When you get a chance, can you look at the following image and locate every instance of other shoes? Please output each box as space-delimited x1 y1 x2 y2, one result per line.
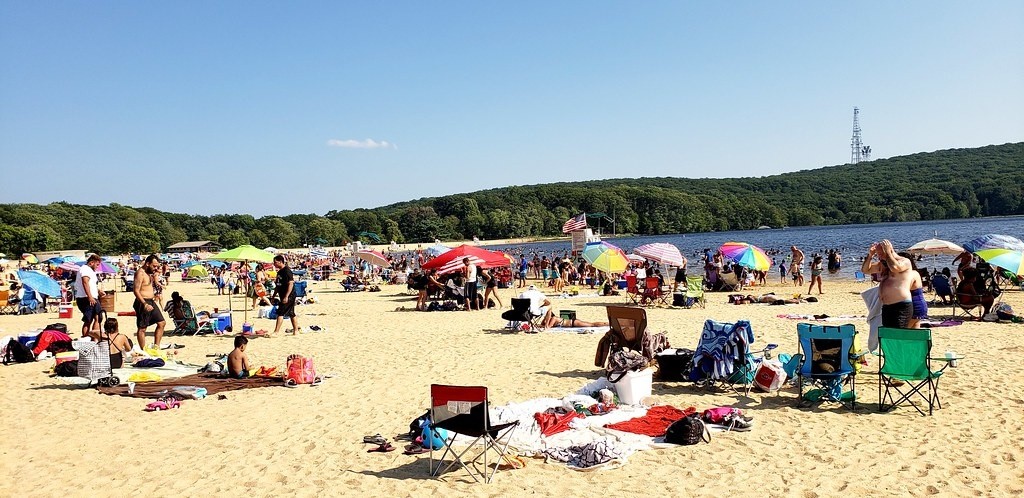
363 433 387 443
394 433 411 441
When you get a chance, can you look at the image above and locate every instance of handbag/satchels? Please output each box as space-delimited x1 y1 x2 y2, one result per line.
703 408 742 423
607 363 654 405
54 360 78 376
284 354 317 384
754 360 787 392
663 413 712 445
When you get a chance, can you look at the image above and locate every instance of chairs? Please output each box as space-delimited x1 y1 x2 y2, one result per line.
680 319 778 398
428 384 520 484
953 293 983 322
295 281 307 306
594 305 671 367
872 327 966 416
339 281 358 292
932 275 955 306
48 289 63 314
121 277 133 292
778 323 869 414
164 300 219 337
996 267 1024 290
684 276 705 308
0 290 39 315
502 298 542 333
626 276 671 305
719 271 740 292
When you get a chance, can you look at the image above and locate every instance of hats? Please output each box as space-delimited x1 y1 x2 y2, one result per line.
528 285 537 290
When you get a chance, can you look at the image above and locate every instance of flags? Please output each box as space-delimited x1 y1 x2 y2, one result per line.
562 212 586 233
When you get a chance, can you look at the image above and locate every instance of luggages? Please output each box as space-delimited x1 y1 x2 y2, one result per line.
72 309 120 387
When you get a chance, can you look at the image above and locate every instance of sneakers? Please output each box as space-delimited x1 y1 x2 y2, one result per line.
722 412 754 431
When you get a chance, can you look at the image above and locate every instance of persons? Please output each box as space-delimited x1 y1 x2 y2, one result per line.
75 253 107 338
0 259 139 314
157 244 287 334
85 311 265 380
288 238 1024 335
134 256 166 349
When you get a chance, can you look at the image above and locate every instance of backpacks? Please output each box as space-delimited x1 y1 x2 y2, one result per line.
3 340 35 365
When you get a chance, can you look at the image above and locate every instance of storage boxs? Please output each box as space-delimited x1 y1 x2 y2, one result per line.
613 280 627 290
56 351 79 365
58 302 73 318
673 292 692 306
17 331 43 348
585 277 598 285
610 367 652 405
211 312 231 332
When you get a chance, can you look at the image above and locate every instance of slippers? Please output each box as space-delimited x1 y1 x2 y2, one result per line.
368 442 395 452
285 379 299 388
404 443 431 454
311 374 323 385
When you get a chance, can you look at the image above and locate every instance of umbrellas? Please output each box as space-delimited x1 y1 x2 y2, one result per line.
582 241 629 274
633 242 683 267
422 243 515 288
717 241 773 271
905 234 1024 277
17 252 120 297
354 248 393 269
206 245 287 323
